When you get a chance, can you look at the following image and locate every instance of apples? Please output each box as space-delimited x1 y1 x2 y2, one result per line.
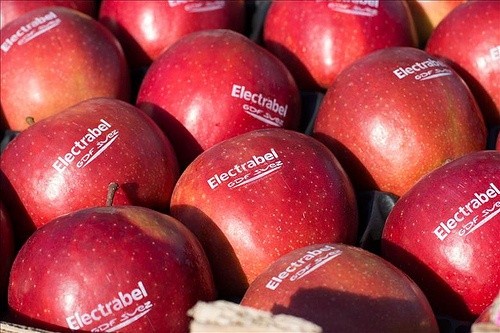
0 0 500 333
